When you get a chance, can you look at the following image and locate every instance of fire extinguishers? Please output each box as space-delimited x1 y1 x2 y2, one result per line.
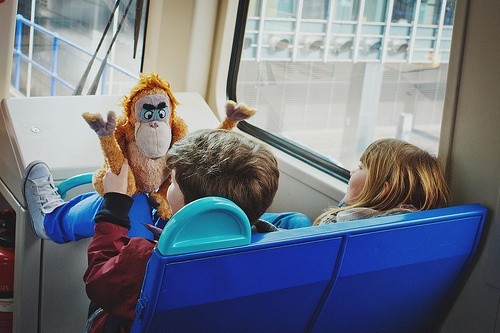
1 210 13 333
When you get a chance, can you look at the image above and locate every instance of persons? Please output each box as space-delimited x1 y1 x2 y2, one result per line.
21 130 281 333
252 138 449 238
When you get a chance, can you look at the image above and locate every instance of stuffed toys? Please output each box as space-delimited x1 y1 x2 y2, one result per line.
82 76 259 197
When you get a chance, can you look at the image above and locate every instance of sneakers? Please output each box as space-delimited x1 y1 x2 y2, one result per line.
21 160 65 240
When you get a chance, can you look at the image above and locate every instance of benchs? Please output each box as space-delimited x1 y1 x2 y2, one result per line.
129 196 488 333
